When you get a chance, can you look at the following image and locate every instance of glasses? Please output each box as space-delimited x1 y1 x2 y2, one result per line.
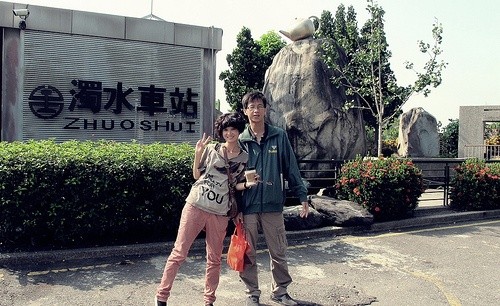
247 105 264 111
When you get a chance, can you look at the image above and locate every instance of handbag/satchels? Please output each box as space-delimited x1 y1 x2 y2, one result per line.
230 187 244 217
227 222 248 272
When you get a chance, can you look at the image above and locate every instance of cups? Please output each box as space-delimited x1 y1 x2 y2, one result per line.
245 170 257 186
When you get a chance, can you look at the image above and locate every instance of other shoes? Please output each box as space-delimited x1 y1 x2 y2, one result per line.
155 295 167 306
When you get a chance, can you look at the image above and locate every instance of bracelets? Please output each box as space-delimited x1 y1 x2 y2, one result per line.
244 182 251 189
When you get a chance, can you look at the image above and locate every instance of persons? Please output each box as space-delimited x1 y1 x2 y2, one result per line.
231 91 309 306
156 113 259 306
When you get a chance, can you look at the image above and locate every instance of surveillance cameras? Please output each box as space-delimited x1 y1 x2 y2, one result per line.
13 9 29 16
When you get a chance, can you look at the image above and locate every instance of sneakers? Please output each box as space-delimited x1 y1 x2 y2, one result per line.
246 296 259 306
270 293 298 306
